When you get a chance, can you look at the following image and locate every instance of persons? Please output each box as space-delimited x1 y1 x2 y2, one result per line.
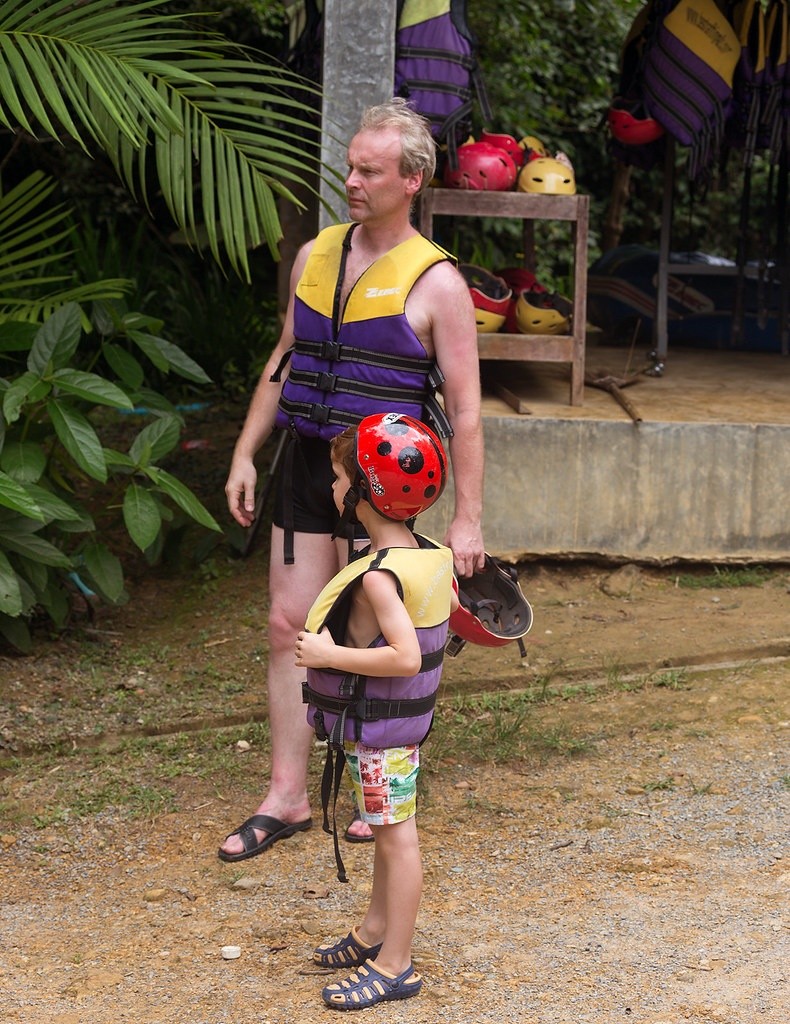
215 98 417 862
293 413 455 1010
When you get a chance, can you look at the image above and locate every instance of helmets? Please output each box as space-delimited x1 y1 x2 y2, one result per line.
453 263 572 334
427 128 577 197
609 99 663 145
450 550 532 649
356 413 448 522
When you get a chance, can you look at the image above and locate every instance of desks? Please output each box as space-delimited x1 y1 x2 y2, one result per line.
417 185 591 407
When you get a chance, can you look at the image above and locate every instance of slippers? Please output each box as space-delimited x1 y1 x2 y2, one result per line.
219 811 313 863
344 803 378 841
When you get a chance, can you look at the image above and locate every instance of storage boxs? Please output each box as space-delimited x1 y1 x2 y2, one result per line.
667 249 738 350
742 259 782 353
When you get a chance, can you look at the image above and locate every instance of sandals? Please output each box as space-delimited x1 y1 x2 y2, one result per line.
322 959 423 1008
313 925 384 968
